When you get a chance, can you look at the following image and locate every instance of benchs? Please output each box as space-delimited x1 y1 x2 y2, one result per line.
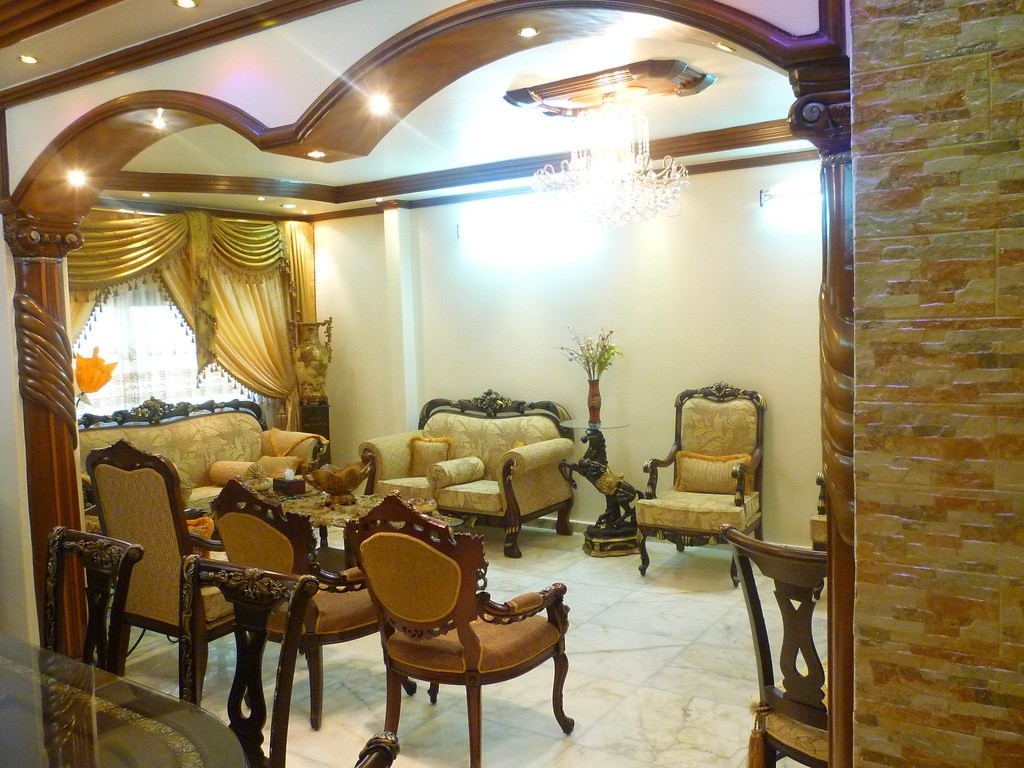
358 389 576 558
78 397 329 513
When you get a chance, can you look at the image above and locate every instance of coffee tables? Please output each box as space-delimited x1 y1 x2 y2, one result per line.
265 492 464 547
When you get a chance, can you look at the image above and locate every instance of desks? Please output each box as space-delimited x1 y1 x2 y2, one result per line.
0 635 246 768
558 419 645 558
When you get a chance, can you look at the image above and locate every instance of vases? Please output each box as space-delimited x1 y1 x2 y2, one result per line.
587 380 601 423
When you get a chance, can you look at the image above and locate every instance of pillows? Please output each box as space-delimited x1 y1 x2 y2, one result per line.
406 435 454 477
674 450 752 495
255 454 302 478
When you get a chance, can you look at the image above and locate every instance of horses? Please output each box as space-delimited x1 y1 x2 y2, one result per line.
558 424 644 530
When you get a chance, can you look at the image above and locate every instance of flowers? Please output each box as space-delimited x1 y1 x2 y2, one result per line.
555 325 624 380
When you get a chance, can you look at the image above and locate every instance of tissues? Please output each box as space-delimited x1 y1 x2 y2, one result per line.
273 468 306 495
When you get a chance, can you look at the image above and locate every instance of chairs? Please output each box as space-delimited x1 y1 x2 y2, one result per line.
210 478 379 730
85 438 234 708
636 381 767 587
721 524 828 768
41 526 144 678
343 492 575 768
